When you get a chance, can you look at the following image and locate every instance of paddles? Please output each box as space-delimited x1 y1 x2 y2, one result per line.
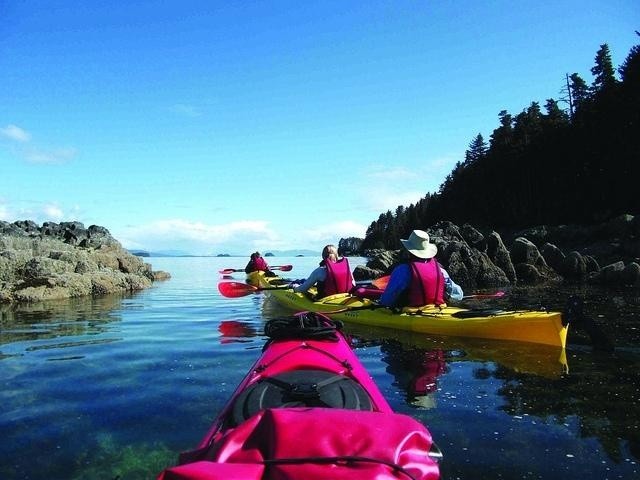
218 275 392 298
218 265 293 274
320 292 504 314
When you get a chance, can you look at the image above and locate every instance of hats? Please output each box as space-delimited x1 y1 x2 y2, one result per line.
400 230 438 259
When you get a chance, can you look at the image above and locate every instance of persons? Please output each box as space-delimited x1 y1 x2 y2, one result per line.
244 251 270 274
380 344 448 410
292 244 356 297
380 229 464 307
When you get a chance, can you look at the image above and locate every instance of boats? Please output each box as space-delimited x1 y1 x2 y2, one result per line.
260 295 571 378
247 270 290 289
157 310 445 478
258 275 572 349
